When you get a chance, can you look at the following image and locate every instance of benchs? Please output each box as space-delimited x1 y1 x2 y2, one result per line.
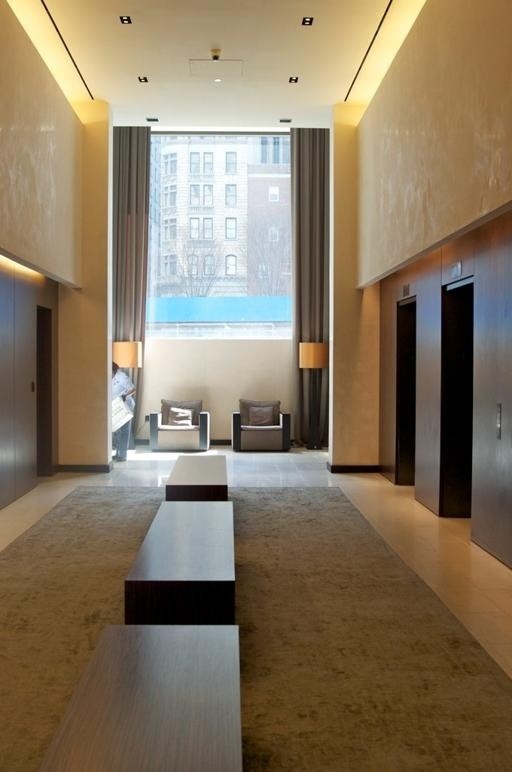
123 499 237 625
42 623 241 772
163 455 229 501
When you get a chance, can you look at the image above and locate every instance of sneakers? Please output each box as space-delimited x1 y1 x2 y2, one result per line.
112 454 127 462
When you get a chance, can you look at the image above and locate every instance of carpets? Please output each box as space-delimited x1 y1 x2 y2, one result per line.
2 485 509 772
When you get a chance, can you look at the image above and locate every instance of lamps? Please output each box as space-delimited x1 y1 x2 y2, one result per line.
113 341 142 378
298 342 328 450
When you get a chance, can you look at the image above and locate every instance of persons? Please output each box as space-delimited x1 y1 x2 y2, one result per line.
110 361 138 463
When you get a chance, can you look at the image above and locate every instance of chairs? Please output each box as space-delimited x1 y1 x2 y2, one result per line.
149 398 291 451
